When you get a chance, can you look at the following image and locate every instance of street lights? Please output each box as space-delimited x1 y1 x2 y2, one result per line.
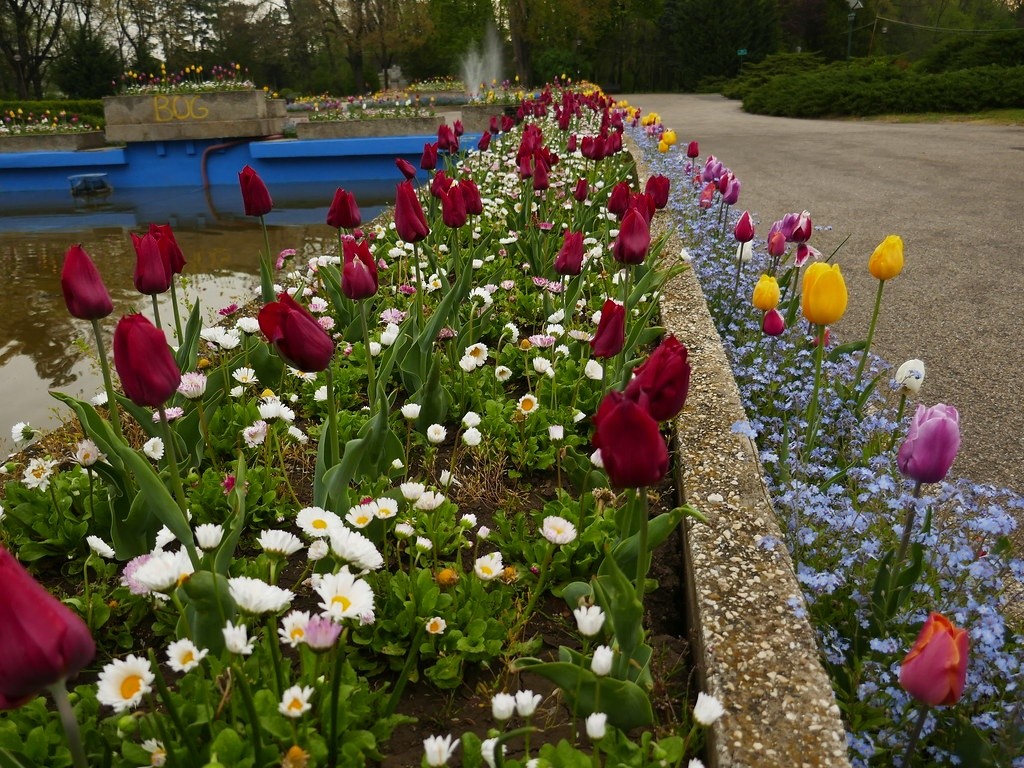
847 13 856 57
